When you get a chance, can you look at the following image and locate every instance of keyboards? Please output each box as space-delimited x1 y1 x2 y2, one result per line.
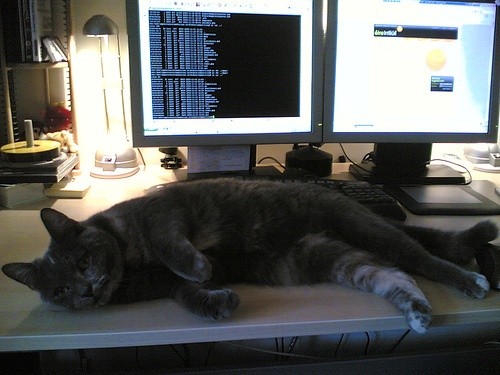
313 179 406 221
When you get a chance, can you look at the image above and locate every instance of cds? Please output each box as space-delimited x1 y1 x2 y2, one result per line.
0 139 68 169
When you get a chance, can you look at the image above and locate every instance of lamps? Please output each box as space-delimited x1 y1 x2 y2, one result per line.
84 15 139 179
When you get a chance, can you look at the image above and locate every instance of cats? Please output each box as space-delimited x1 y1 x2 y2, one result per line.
1 176 500 335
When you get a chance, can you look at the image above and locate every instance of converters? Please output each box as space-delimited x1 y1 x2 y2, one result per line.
251 166 283 182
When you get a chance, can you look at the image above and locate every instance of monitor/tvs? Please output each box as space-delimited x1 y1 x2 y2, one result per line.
125 0 323 180
324 0 499 184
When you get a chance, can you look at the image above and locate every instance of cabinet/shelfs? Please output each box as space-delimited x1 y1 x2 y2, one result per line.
0 3 83 190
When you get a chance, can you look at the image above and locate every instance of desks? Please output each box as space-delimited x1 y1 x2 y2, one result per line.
1 161 500 354
